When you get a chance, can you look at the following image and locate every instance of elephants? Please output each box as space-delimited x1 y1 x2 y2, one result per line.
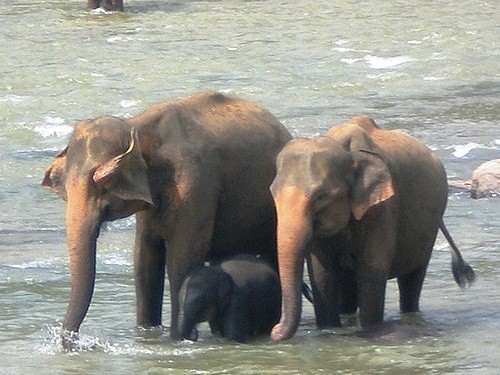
267 115 476 342
43 92 293 348
172 249 284 341
